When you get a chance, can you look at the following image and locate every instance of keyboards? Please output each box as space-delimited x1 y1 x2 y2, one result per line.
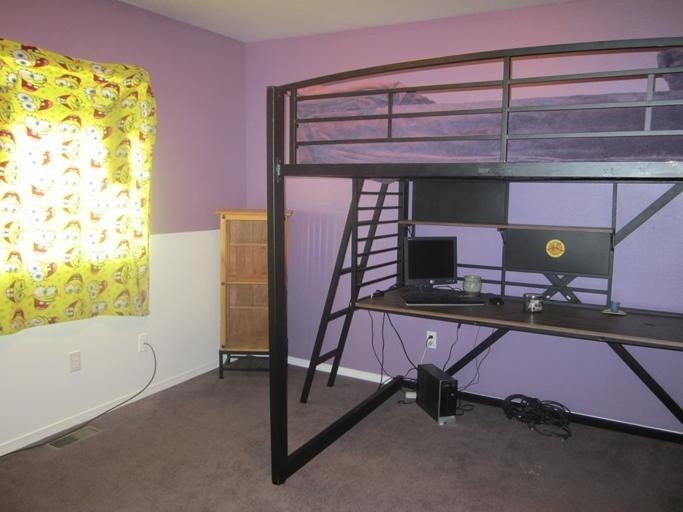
401 295 485 307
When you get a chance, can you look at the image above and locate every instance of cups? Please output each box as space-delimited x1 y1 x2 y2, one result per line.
463 274 481 295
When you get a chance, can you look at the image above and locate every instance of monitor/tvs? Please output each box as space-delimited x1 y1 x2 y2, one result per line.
403 236 457 294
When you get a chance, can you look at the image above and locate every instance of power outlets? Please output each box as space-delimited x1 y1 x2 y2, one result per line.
427 331 437 349
139 334 151 352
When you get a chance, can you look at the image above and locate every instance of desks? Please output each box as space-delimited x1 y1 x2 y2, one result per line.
356 286 683 354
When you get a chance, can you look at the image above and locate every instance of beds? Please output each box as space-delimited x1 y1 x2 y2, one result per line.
267 39 683 485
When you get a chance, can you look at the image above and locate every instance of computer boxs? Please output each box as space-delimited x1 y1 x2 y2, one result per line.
416 363 458 426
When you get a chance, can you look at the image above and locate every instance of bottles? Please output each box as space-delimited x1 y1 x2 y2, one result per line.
524 293 544 313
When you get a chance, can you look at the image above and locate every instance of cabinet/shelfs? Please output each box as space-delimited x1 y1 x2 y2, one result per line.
213 207 294 382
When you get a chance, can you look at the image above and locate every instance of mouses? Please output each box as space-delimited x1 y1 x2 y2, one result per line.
489 296 503 306
